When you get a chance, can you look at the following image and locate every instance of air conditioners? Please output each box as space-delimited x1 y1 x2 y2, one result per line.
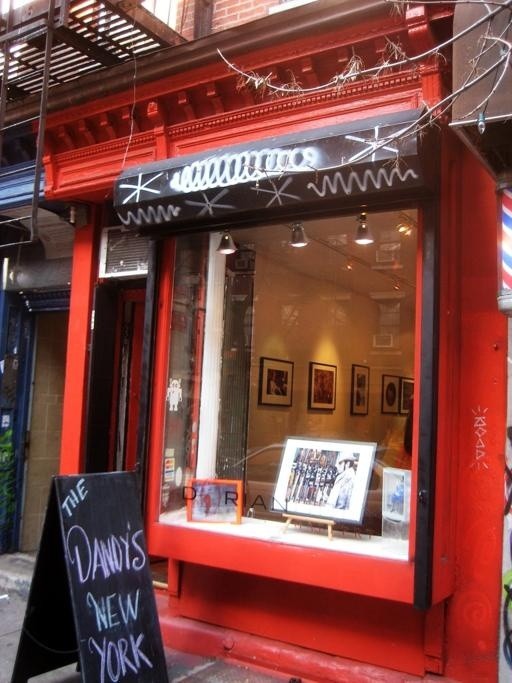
376 250 394 262
373 334 394 348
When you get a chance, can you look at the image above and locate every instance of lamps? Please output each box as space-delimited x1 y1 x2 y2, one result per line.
289 221 309 249
354 212 374 245
216 230 239 255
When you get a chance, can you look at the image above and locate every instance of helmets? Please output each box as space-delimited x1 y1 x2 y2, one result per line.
336 452 356 465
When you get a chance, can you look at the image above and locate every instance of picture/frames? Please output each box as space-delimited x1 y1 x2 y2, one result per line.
308 361 337 410
269 435 379 526
258 357 294 407
382 374 414 416
351 364 370 416
186 478 242 525
382 466 411 524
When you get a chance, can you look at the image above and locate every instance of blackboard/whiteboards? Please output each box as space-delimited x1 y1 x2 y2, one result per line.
54 469 168 683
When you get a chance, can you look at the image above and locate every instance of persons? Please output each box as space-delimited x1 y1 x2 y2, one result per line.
294 449 357 510
387 476 405 513
204 489 212 515
271 371 281 395
279 377 288 395
